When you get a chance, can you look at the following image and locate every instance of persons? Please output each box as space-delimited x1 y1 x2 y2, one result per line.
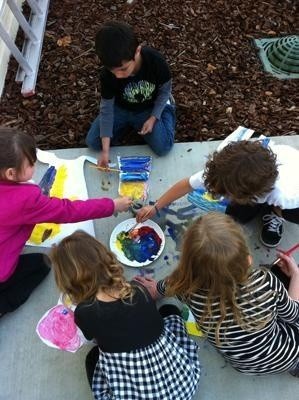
51 231 201 398
132 214 299 377
1 127 132 317
135 138 299 247
84 21 175 172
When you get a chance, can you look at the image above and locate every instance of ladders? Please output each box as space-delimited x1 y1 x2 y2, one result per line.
0 0 49 98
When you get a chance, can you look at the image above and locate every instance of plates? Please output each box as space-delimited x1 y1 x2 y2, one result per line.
109 216 167 268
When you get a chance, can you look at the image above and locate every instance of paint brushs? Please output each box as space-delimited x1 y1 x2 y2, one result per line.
125 202 158 236
88 164 126 173
130 200 141 208
272 243 299 266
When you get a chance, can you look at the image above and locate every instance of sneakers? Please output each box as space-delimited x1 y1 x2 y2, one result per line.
259 210 286 248
